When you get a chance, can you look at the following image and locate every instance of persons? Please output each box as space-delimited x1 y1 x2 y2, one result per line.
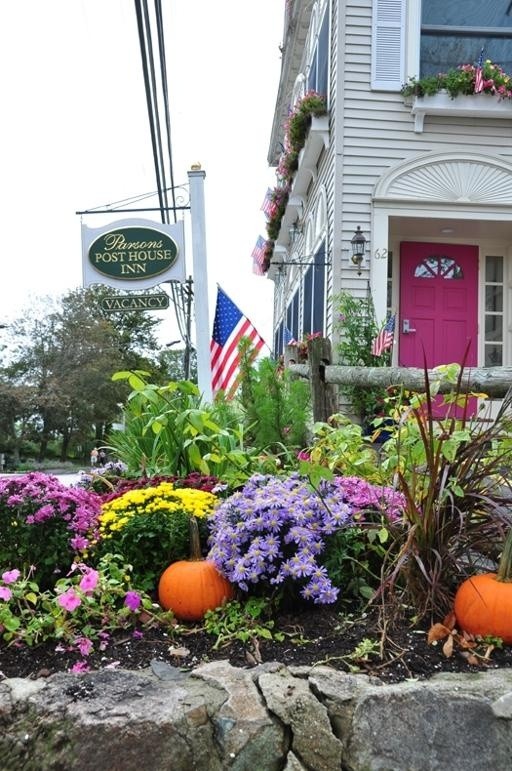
98 448 107 465
90 445 99 468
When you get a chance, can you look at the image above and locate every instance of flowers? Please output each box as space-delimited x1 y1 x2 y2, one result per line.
254 87 325 276
398 60 512 105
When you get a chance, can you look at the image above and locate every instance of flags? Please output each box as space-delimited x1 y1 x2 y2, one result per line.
369 314 395 357
209 280 271 407
473 46 486 95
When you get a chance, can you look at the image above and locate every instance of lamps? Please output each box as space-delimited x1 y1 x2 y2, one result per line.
350 224 367 276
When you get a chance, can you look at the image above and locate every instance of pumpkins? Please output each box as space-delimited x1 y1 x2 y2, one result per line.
455 534 512 647
158 517 235 622
